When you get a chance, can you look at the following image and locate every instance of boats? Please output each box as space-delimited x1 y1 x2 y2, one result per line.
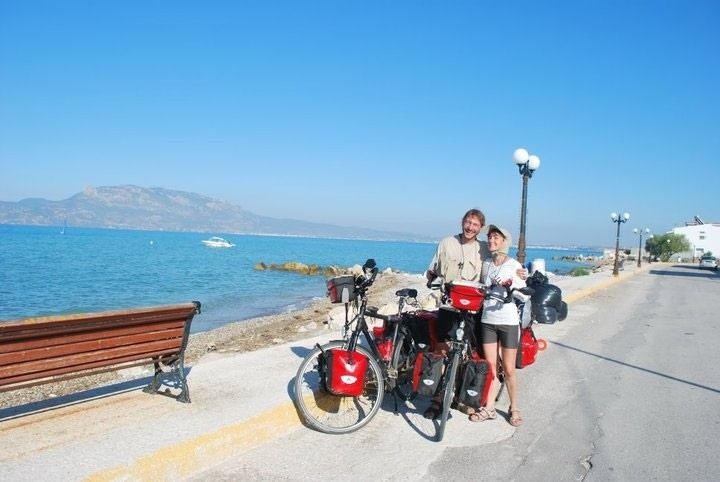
201 237 234 248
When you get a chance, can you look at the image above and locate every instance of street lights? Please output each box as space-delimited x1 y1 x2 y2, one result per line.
611 212 630 274
634 227 654 266
514 148 540 267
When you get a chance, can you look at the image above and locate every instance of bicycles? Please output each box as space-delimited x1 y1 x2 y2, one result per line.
430 272 522 441
295 258 430 435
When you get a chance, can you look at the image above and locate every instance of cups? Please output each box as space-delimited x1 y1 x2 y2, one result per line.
376 336 393 361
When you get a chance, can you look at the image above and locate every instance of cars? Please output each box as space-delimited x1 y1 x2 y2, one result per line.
698 255 717 270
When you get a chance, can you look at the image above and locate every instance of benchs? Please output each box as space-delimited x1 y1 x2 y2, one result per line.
0 298 204 405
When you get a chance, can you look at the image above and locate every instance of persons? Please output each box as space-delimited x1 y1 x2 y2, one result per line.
420 208 529 310
468 223 526 427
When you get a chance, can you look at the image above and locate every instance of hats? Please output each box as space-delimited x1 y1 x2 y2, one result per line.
485 224 512 258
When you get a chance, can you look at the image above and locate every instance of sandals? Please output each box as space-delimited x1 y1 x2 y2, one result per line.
507 406 523 427
469 408 498 423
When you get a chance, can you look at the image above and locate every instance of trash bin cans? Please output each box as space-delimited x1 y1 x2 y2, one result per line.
529 259 546 276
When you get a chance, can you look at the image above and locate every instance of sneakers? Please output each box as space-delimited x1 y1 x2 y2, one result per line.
423 407 439 420
451 401 475 416
